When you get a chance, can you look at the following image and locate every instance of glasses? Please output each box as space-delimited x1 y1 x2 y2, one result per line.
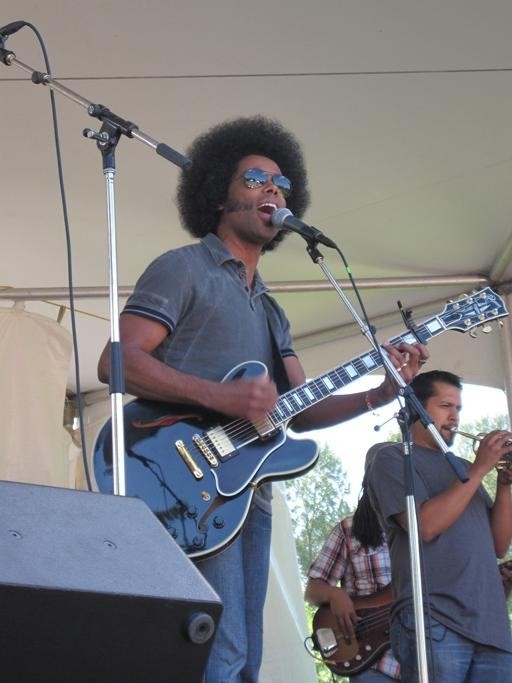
231 168 293 198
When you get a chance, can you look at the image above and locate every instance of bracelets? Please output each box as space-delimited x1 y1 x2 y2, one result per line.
365 389 375 411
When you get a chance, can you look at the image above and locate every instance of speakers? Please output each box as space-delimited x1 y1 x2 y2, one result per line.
0 480 224 683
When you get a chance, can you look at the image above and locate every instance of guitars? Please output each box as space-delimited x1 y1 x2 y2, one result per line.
93 286 508 562
311 562 511 676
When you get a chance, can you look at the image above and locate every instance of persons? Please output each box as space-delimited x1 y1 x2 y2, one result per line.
97 116 429 682
303 440 512 683
367 370 512 683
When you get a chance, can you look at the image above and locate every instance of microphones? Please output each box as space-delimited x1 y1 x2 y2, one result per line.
271 207 338 249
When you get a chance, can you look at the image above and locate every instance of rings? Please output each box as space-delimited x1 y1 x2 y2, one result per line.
396 367 402 371
418 359 425 363
401 362 407 367
